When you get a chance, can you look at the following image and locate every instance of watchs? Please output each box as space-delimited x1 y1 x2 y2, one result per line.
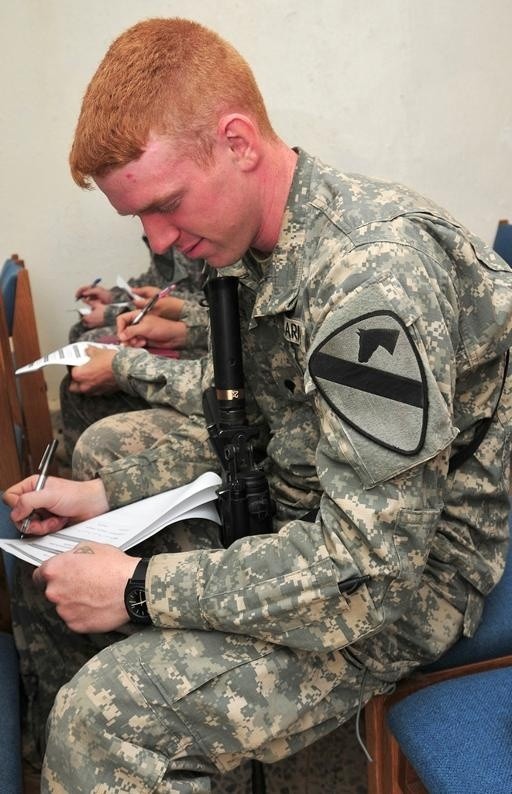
124 557 153 627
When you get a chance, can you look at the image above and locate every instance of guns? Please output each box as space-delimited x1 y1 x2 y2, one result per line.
202 275 276 792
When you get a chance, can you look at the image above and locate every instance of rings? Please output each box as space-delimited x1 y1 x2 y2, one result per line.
77 384 81 393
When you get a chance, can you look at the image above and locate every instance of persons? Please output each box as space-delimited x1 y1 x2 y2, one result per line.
56 235 215 482
0 17 512 794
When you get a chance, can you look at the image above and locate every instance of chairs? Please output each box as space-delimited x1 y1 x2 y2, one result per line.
358 218 512 794
0 254 59 791
